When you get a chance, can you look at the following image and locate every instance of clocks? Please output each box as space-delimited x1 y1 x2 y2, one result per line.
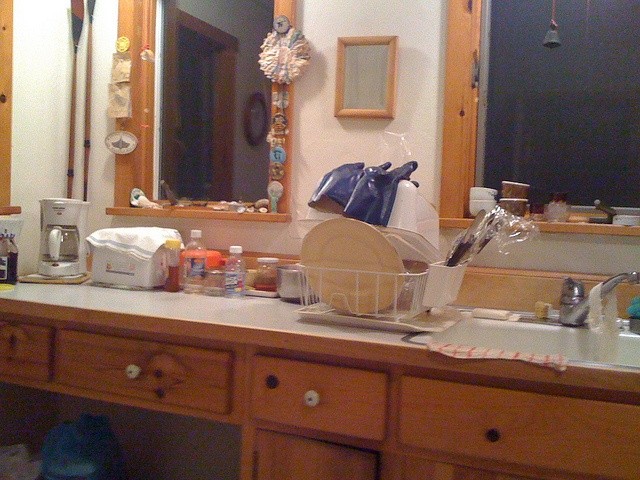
245 94 267 146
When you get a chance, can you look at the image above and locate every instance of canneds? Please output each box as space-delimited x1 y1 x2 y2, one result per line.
254 257 279 291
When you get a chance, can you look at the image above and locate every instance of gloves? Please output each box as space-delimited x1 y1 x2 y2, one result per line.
343 161 419 228
308 162 365 207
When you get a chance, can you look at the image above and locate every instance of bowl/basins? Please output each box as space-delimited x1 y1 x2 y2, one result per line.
277 266 318 302
470 187 496 201
468 201 497 219
500 200 527 218
500 181 529 199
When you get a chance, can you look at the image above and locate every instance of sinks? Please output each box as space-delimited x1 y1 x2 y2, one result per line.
430 316 640 367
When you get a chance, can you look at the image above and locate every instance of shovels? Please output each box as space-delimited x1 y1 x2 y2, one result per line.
446 207 486 271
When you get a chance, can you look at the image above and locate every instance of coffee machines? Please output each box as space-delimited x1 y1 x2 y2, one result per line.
37 198 88 278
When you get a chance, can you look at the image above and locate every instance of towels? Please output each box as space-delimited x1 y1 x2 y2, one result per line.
84 225 185 260
422 337 570 374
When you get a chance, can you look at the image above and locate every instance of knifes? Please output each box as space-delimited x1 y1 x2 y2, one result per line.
448 208 506 266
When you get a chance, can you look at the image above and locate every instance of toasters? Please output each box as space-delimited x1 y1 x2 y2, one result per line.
90 229 169 287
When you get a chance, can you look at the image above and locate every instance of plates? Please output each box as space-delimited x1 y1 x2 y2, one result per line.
301 217 403 313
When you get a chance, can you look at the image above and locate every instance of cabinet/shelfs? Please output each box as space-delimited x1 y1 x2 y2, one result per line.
55 306 246 428
391 344 640 480
0 299 55 393
241 327 391 480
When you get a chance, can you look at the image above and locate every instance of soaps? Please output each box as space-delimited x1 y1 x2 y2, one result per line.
470 306 510 319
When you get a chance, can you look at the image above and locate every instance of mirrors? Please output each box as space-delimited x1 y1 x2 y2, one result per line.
334 36 397 119
105 0 296 225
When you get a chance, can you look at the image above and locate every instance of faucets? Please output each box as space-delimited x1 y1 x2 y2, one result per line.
559 272 637 326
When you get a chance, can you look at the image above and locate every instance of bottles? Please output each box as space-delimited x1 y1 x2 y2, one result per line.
224 245 247 296
180 229 206 293
164 240 181 292
0 233 17 285
